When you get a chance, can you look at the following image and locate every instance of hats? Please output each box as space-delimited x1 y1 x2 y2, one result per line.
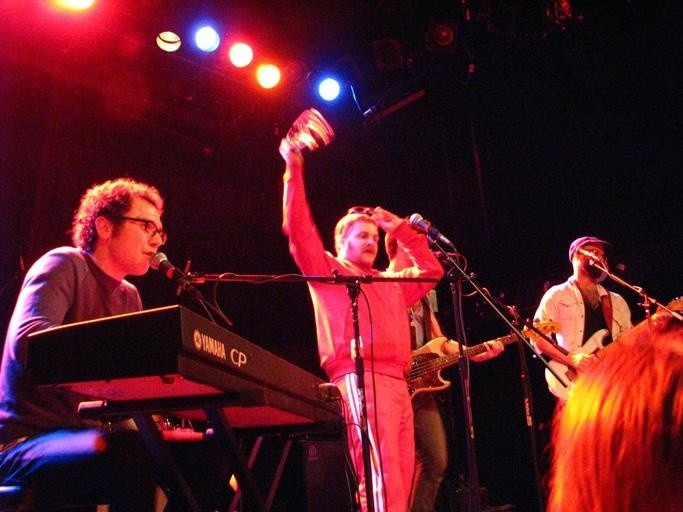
568 235 615 264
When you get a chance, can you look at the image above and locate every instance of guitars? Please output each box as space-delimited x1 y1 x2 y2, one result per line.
407 318 563 401
545 295 683 400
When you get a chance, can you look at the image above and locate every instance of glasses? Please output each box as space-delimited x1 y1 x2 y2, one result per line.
116 214 167 247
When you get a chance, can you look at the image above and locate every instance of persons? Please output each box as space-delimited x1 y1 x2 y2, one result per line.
546 305 683 512
0 177 230 511
277 132 444 512
530 235 632 424
384 214 504 511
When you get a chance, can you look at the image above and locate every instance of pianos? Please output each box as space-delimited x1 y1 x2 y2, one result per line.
25 304 340 442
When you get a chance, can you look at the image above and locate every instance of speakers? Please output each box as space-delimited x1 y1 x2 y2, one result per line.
302 438 348 512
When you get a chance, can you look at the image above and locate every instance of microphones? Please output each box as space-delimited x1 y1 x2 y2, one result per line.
149 252 204 301
409 213 452 249
573 245 600 261
